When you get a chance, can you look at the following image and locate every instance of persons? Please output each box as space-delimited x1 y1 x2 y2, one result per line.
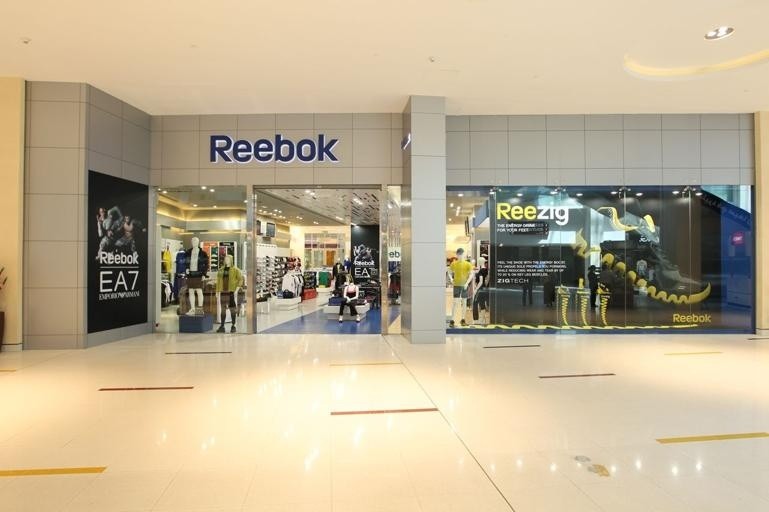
107 215 147 253
541 263 555 307
472 257 488 325
521 263 534 306
599 264 617 291
215 254 244 333
96 205 124 261
184 236 210 316
338 282 361 322
448 248 474 328
587 265 599 310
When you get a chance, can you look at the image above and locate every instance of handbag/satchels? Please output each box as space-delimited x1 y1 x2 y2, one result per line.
283 292 293 298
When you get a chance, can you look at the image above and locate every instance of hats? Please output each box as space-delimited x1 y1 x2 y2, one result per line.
456 249 463 256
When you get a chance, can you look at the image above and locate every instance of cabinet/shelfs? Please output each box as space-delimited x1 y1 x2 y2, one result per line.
199 242 237 271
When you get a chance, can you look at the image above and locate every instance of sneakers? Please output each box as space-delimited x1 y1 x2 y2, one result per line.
231 327 236 332
217 327 226 332
461 319 465 326
449 320 454 327
339 315 343 322
355 315 360 322
186 310 194 316
211 253 218 267
